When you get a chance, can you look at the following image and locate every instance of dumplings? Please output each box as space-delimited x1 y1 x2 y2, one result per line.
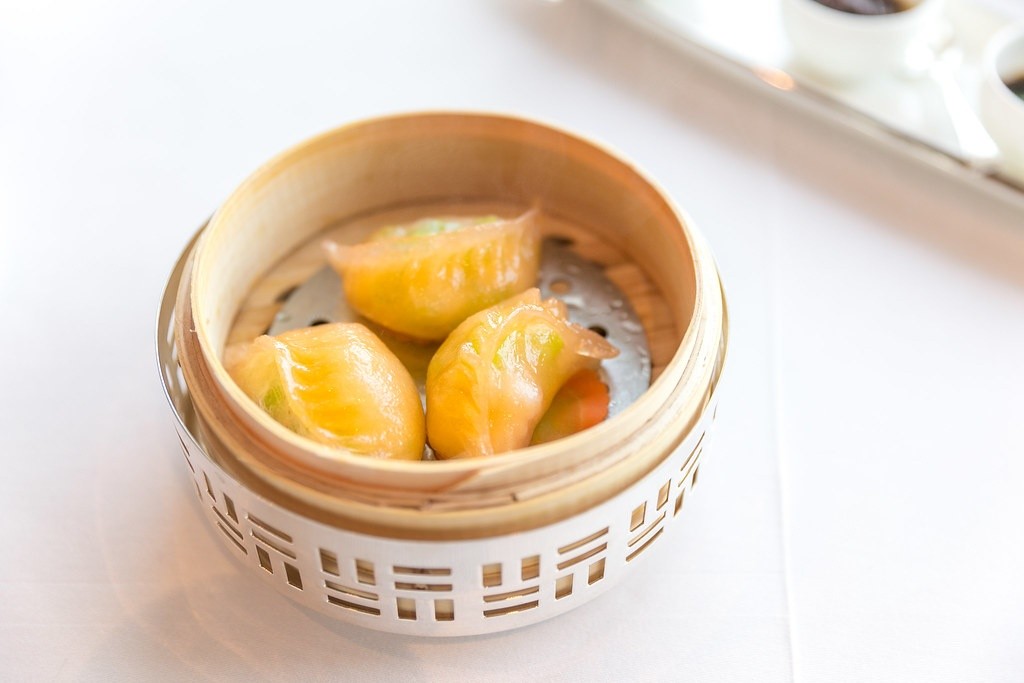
220 199 619 462
